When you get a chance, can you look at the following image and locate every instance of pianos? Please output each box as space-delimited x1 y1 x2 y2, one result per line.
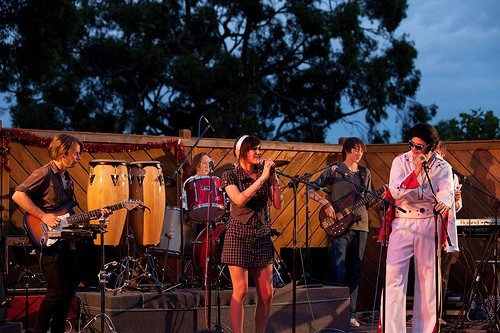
455 217 500 333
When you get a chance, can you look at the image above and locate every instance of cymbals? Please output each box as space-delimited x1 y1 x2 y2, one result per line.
164 176 177 188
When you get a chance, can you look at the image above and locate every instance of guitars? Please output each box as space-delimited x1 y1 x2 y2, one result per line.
318 183 389 238
23 198 144 250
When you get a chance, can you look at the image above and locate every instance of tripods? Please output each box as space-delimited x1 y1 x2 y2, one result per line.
288 161 346 287
157 126 210 294
81 232 118 333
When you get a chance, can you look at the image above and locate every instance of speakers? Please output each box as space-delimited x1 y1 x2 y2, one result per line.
446 233 497 303
0 235 47 294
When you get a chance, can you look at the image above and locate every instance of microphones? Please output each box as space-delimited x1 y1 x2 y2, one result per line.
260 159 277 170
421 156 430 170
452 168 469 182
208 158 214 173
331 165 348 176
204 117 214 132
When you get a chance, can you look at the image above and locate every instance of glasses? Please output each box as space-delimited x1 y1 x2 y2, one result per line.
409 139 433 151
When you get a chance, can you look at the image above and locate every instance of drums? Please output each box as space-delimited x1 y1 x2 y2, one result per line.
192 223 231 287
181 175 226 222
86 159 129 246
128 161 165 247
148 206 181 256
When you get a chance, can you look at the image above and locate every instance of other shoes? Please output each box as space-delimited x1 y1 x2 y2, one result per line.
439 318 447 324
349 318 359 327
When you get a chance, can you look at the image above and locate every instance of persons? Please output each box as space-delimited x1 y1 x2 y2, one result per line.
12 134 113 333
379 122 456 333
304 137 385 330
220 135 281 333
432 141 463 328
180 152 214 256
